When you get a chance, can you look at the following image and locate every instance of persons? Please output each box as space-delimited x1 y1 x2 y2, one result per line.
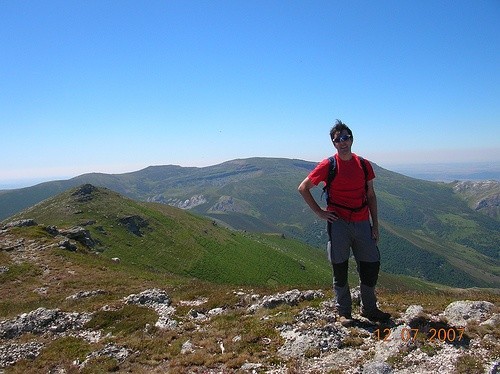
298 119 391 324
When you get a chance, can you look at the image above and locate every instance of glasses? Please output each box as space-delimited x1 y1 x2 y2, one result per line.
332 135 351 143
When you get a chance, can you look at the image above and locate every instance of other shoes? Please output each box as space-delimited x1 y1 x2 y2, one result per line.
360 306 391 320
336 305 352 317
